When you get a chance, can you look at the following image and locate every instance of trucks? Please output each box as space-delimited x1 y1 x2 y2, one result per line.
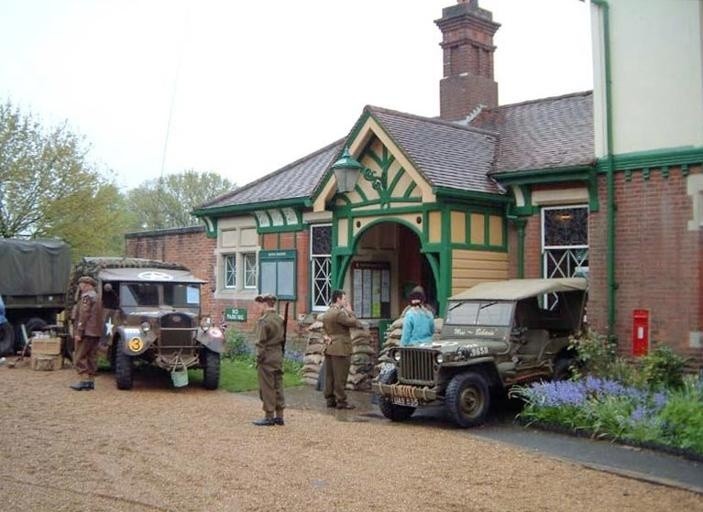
0 237 73 355
61 256 225 390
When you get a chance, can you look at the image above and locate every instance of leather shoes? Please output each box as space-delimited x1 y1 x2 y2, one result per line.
252 417 283 426
328 401 356 409
69 381 95 392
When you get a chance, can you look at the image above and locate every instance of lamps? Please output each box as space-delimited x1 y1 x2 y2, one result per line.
330 144 382 196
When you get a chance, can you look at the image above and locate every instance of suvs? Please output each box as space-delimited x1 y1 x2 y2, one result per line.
370 276 589 429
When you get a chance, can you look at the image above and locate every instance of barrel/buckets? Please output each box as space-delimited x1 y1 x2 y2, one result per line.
170 355 188 387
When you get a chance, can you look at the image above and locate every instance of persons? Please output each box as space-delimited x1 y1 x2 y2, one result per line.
400 293 435 346
322 289 356 409
400 285 437 316
0 296 12 362
251 293 285 426
70 276 104 390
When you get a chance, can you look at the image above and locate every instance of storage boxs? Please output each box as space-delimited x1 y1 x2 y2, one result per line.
29 335 63 370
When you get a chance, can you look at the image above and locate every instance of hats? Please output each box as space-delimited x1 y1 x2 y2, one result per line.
254 294 275 301
77 276 96 286
